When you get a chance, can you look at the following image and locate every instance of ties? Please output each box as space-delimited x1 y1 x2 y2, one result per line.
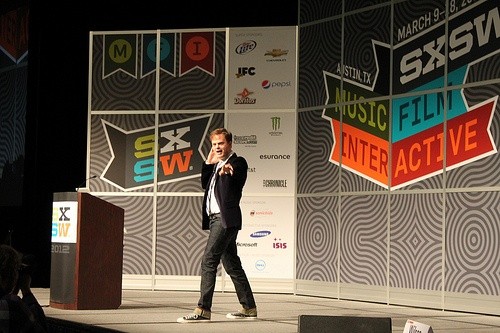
206 161 222 216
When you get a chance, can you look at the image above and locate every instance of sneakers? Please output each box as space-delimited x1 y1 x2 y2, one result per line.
226 312 257 319
177 313 211 321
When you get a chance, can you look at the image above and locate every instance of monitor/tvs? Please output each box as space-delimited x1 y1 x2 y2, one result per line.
76 188 90 194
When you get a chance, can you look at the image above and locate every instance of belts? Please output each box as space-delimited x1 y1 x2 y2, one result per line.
209 214 222 219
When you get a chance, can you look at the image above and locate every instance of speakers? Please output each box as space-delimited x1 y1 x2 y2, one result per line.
298 314 392 333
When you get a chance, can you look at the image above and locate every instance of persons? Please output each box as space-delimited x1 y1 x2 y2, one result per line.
176 128 257 323
0 244 50 333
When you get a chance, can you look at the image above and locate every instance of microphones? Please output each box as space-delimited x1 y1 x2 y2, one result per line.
77 175 97 192
